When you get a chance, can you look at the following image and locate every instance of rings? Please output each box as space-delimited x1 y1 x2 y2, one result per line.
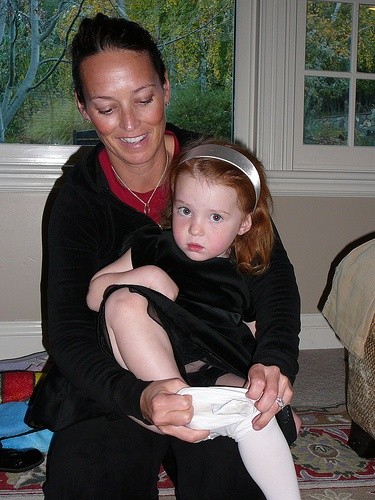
275 397 284 411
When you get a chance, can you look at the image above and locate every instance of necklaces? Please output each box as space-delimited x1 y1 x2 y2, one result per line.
111 155 168 215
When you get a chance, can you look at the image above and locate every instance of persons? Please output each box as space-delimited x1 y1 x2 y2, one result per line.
25 16 302 499
87 139 299 499
